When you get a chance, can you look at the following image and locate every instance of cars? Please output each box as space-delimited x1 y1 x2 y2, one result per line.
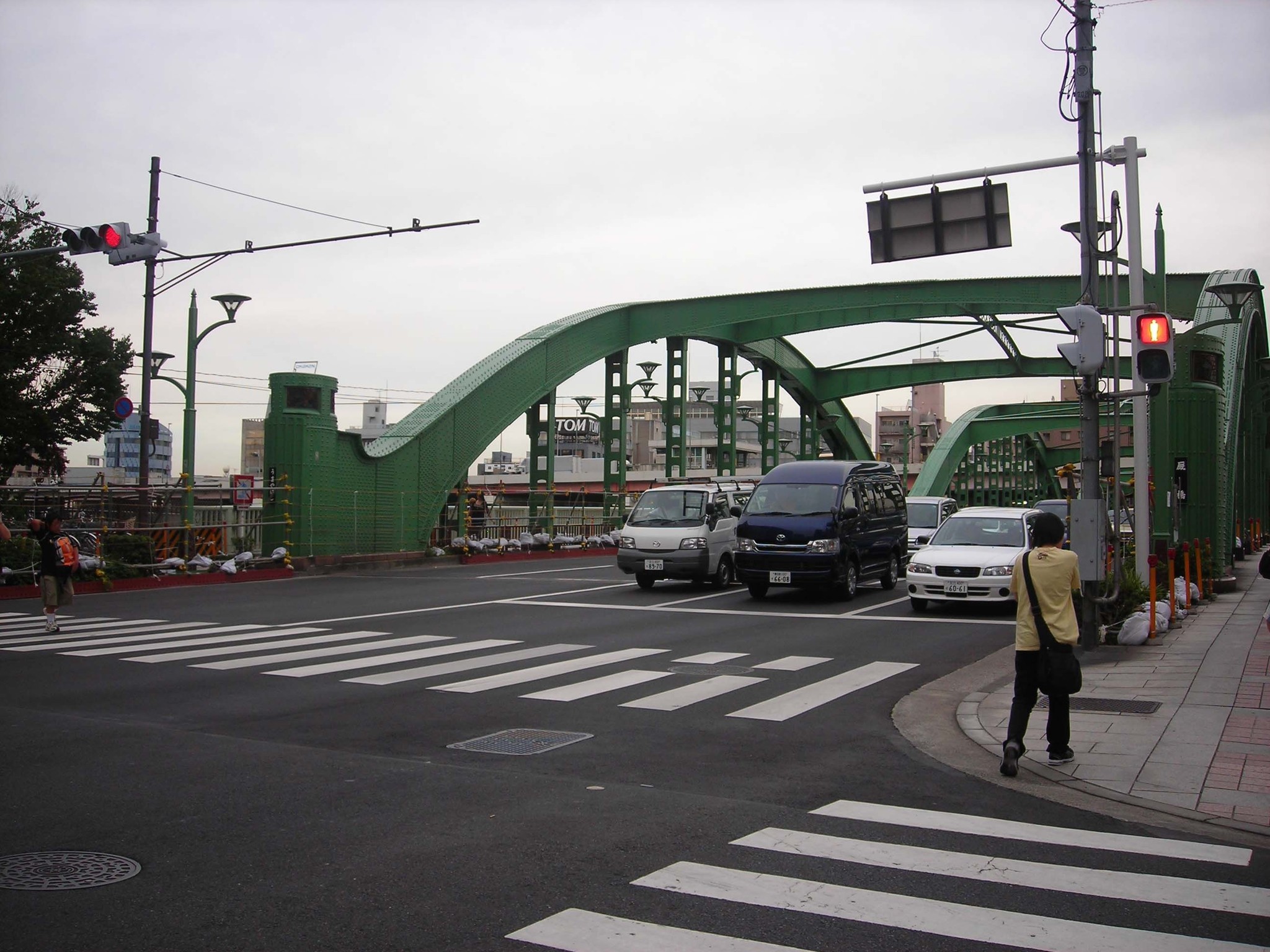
904 505 1046 612
1031 498 1073 550
1107 507 1135 557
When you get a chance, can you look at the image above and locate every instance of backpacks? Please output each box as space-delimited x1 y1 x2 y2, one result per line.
49 530 78 568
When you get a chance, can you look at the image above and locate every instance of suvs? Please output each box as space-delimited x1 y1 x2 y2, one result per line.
906 496 959 561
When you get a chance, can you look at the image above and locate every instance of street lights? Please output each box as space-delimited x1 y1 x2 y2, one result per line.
876 394 880 461
1058 190 1125 605
637 380 688 477
778 404 843 461
736 405 780 476
570 360 663 537
134 286 253 573
879 421 931 497
689 386 736 476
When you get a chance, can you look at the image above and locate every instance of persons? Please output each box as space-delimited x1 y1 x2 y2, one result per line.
31 511 79 632
466 491 495 540
998 512 1082 778
0 512 11 541
768 490 795 510
646 495 680 518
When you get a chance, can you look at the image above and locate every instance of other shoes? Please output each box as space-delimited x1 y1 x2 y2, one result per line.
999 741 1020 777
1048 747 1074 766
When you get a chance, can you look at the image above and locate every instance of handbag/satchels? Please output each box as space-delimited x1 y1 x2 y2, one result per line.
1035 637 1082 694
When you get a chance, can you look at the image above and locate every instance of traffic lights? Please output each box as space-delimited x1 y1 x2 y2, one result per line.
1136 311 1174 384
1056 303 1105 376
62 221 131 256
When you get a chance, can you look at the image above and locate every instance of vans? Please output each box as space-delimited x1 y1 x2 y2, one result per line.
617 473 765 591
728 458 909 602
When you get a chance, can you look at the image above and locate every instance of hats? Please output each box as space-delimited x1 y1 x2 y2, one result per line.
1033 512 1065 542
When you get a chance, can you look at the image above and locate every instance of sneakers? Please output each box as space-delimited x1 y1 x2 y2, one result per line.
43 607 56 621
45 622 60 631
